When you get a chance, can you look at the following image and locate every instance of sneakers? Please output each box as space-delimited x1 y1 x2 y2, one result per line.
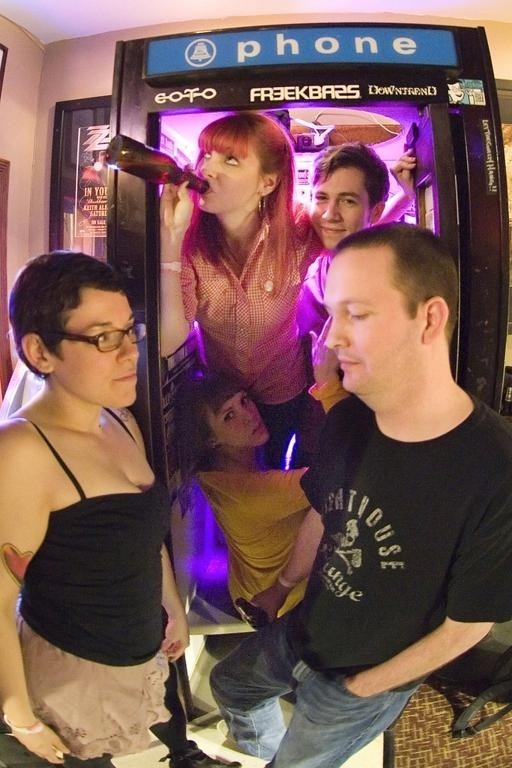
169 741 242 768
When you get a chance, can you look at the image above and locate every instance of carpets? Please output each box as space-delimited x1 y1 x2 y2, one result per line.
394 683 512 768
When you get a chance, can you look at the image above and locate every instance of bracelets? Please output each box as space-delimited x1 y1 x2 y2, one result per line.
2 712 43 735
158 262 183 274
272 568 300 589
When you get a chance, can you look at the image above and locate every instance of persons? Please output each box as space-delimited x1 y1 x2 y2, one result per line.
173 318 348 631
152 117 416 477
291 143 389 474
0 250 241 768
208 223 512 768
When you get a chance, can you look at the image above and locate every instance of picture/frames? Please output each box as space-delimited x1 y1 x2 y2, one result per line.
496 76 511 350
49 93 112 261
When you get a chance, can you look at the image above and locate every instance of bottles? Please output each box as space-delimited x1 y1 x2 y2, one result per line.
233 597 269 632
102 135 209 196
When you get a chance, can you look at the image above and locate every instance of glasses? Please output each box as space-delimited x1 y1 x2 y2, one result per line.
62 322 147 353
